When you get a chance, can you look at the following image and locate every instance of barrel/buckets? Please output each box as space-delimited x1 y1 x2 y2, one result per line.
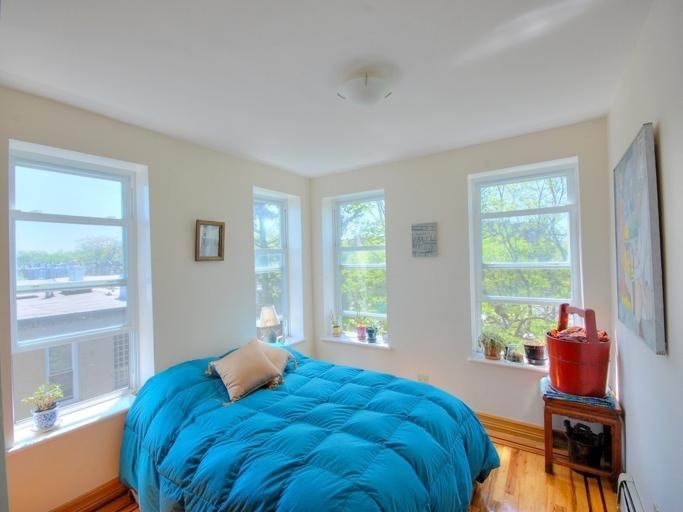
545 303 609 400
565 424 604 478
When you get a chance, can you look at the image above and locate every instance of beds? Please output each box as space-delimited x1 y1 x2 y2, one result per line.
118 343 502 512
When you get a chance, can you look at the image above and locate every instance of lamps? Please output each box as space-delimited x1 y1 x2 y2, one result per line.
258 305 281 343
336 71 398 109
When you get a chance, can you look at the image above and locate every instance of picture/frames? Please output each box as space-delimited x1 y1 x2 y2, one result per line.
195 219 225 262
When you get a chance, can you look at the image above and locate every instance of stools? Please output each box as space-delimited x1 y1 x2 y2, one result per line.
543 388 624 495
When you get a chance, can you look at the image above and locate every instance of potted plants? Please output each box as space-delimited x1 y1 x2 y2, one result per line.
329 309 380 343
476 331 549 365
20 381 64 432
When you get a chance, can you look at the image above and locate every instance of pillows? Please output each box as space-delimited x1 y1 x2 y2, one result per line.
204 336 298 407
